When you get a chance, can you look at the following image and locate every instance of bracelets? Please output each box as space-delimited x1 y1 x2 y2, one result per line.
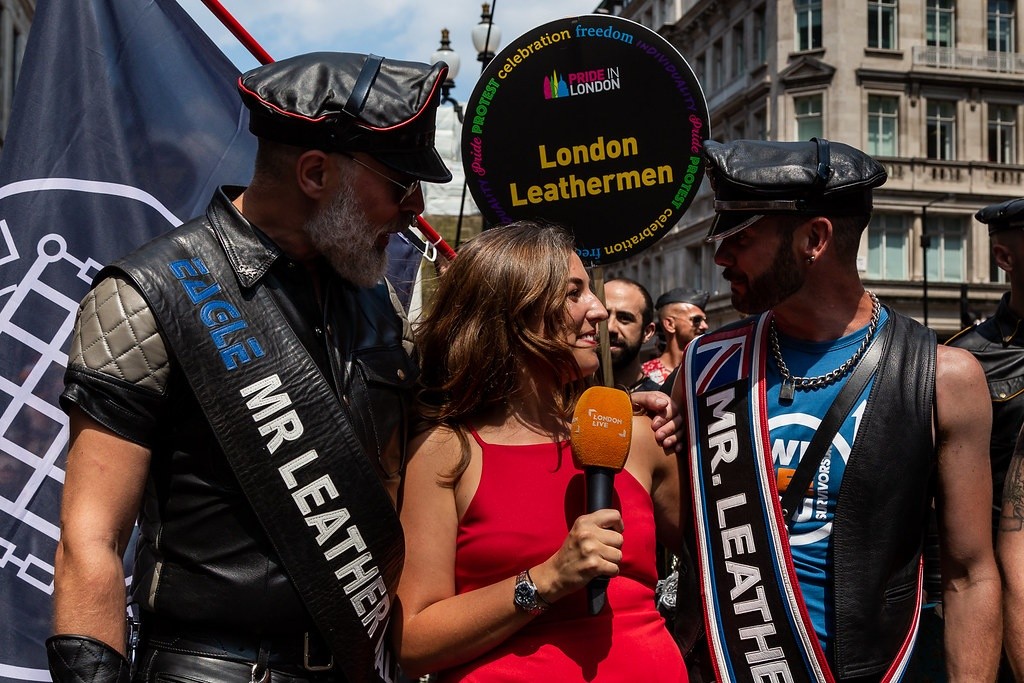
524 567 552 607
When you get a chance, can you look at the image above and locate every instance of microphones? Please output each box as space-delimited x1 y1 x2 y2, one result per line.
570 386 633 615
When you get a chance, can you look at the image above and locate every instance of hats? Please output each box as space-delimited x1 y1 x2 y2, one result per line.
236 50 454 183
655 287 710 311
974 197 1024 236
704 137 887 243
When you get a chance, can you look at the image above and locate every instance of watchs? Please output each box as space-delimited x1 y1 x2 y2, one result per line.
512 572 552 619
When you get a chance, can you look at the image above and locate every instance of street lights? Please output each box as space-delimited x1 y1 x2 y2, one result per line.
432 2 503 237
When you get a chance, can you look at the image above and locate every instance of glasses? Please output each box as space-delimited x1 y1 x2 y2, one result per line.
325 147 421 205
691 314 708 327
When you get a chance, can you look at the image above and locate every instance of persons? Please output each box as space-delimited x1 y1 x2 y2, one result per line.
46 52 1023 682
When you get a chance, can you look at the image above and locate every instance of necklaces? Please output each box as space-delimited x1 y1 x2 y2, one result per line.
771 290 882 401
